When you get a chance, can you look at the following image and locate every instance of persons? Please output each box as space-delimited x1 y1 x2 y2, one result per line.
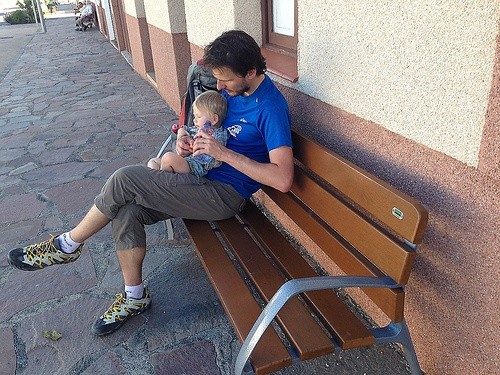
7 29 293 337
73 0 96 32
146 89 227 178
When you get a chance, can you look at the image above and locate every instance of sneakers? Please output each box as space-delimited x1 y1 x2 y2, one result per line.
93 282 151 337
7 234 84 272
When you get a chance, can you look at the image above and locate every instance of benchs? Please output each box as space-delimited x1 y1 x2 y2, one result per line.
156 129 429 375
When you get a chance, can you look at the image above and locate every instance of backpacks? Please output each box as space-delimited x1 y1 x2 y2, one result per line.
170 58 223 155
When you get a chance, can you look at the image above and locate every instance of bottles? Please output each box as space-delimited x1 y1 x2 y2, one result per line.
195 120 216 164
